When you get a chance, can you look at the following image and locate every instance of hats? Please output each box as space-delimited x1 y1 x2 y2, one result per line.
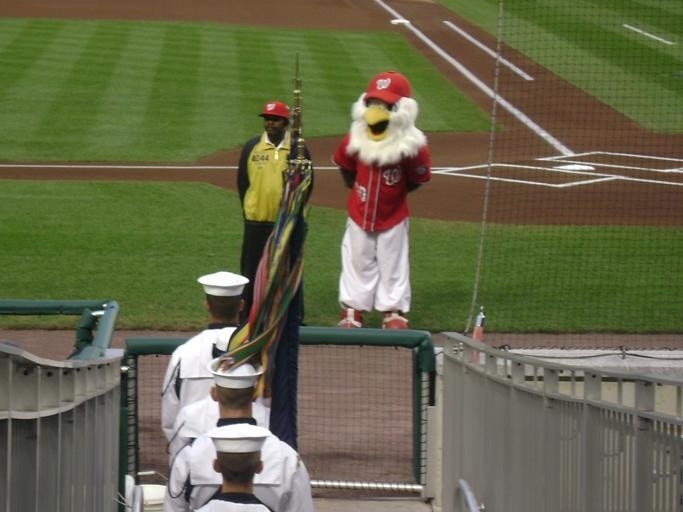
257 100 290 120
361 69 412 106
205 354 266 390
205 422 272 455
195 270 251 298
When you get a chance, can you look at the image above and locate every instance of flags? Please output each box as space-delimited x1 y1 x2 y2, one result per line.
468 314 485 362
215 130 314 450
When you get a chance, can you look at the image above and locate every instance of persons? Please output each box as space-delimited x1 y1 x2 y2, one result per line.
196 423 276 512
160 272 271 440
167 326 270 469
236 102 313 326
164 356 314 512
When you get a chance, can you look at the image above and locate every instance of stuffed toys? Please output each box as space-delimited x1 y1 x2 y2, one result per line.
332 71 431 329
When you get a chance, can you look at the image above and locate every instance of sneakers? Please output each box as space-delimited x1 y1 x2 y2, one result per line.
335 307 364 330
380 309 410 331
